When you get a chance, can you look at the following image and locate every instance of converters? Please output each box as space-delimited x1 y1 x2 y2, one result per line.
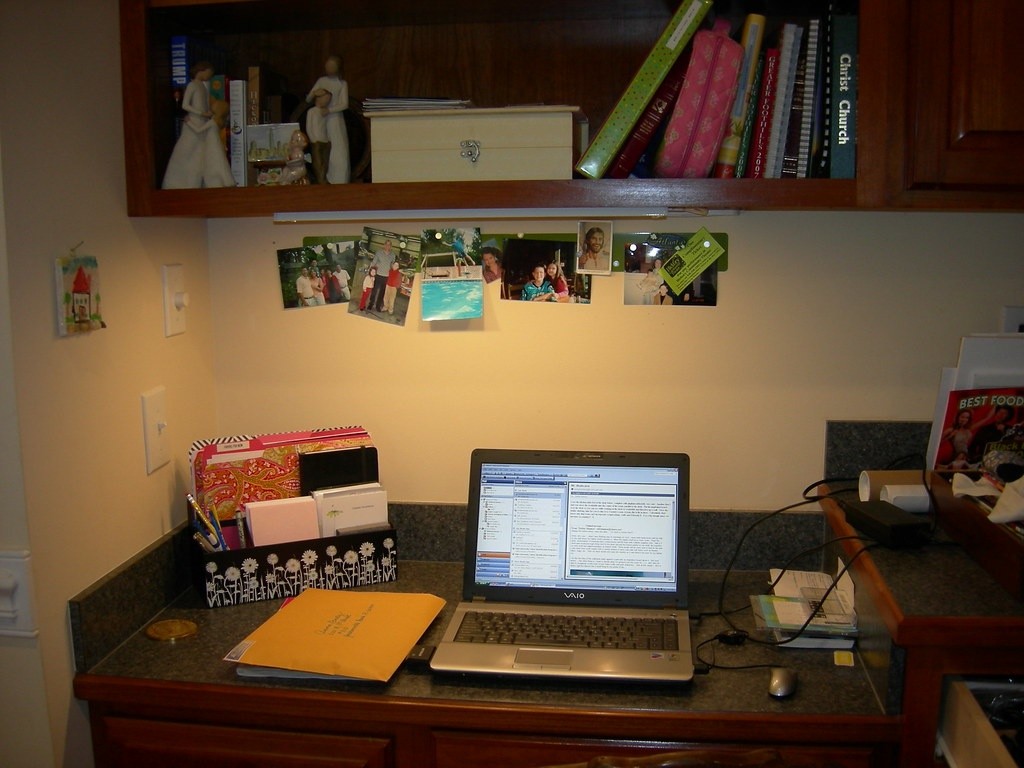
847 500 931 550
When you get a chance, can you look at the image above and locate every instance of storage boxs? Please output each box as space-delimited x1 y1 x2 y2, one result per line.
362 106 590 183
187 494 398 609
931 469 1024 604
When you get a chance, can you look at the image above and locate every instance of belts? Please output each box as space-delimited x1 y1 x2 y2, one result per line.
304 296 313 299
340 287 346 288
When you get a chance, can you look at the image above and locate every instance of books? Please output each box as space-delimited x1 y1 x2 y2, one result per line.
575 0 856 181
236 663 374 684
245 446 389 544
774 632 856 650
169 35 264 187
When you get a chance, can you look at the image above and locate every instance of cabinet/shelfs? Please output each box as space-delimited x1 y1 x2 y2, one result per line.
119 0 1024 219
75 479 1024 768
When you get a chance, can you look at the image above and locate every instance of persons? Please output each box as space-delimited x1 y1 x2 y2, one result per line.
942 402 1012 471
163 56 351 191
296 226 719 314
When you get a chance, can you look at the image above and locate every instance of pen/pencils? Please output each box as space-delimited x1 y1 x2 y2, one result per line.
184 491 247 553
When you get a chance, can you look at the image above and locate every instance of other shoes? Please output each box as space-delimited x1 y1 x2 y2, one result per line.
381 307 387 312
376 306 381 312
388 308 393 314
473 261 475 266
367 305 373 311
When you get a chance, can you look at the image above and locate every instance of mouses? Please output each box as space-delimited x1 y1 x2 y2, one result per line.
769 669 798 697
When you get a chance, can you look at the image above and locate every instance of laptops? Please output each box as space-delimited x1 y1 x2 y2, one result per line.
430 449 693 682
